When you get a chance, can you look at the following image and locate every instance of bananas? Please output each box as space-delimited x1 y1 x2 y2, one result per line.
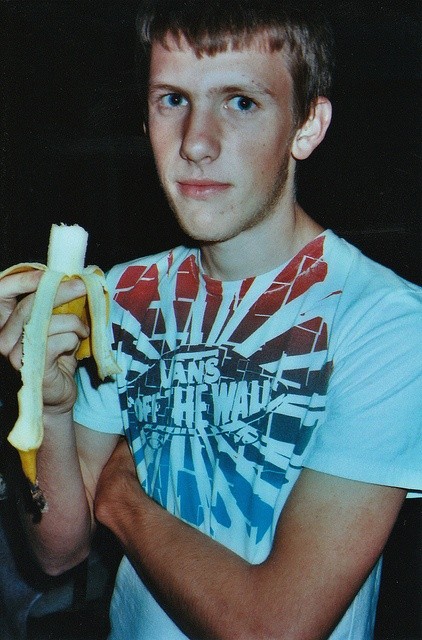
1 222 123 513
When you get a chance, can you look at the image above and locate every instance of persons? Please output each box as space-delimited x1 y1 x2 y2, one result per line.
0 1 422 638
